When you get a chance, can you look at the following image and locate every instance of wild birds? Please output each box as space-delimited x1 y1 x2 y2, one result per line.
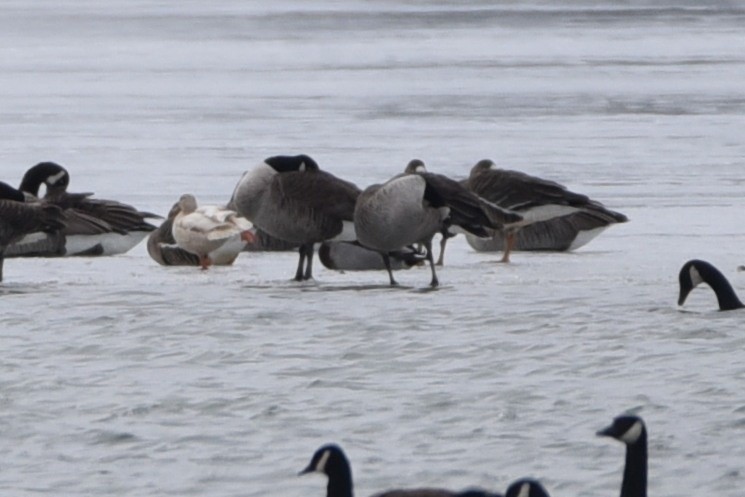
1 148 631 288
677 259 744 310
297 413 647 497
